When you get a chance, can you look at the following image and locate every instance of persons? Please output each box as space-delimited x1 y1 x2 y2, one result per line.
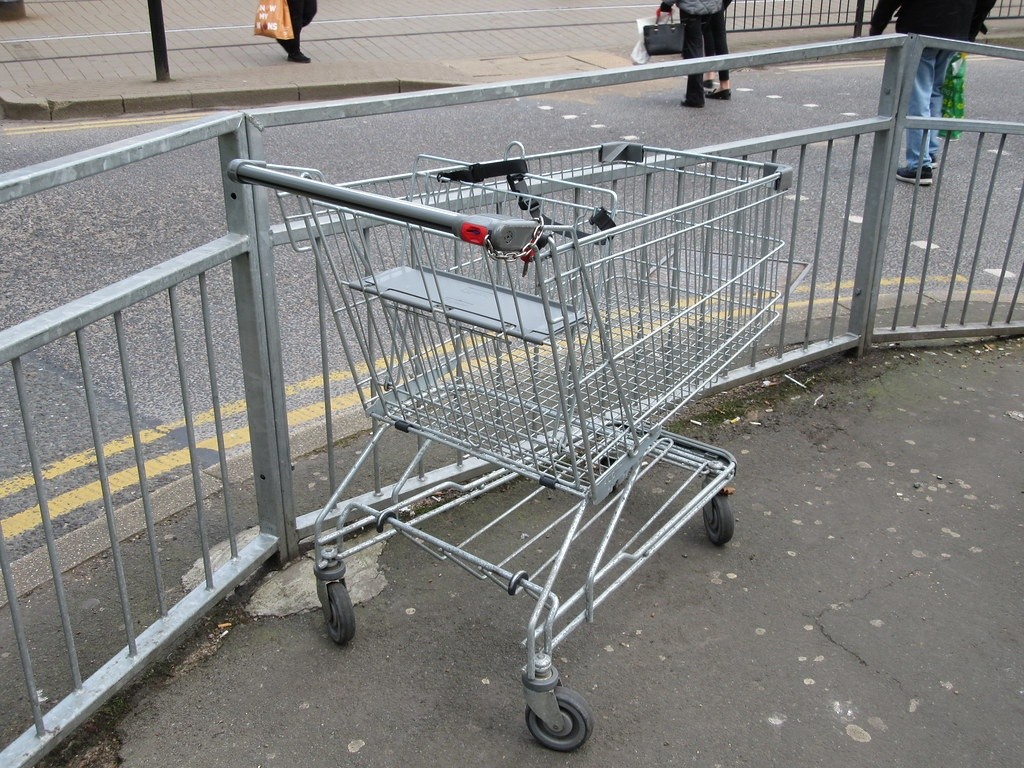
870 0 997 186
658 0 731 108
277 0 318 63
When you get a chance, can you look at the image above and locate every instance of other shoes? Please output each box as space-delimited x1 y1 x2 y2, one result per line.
288 52 311 63
705 88 731 99
703 80 712 88
681 100 704 107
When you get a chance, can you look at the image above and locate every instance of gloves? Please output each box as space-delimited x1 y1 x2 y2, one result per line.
660 2 671 12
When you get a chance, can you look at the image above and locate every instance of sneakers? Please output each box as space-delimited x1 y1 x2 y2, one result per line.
896 165 932 185
930 154 938 168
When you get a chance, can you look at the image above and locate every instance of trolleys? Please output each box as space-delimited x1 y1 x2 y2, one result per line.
228 140 812 752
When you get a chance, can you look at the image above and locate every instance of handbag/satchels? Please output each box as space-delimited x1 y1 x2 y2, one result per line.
254 0 295 40
643 10 685 55
631 8 675 65
937 52 968 140
